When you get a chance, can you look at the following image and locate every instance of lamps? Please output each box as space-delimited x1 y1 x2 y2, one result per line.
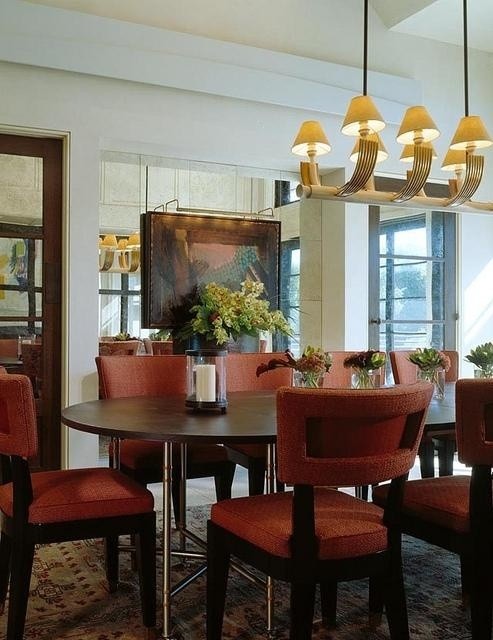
99 234 140 273
291 0 493 215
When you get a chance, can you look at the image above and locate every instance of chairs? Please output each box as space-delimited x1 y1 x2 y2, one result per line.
0 335 176 396
0 374 158 640
321 352 387 500
392 350 459 476
205 352 295 495
204 380 434 640
97 353 239 570
369 375 492 640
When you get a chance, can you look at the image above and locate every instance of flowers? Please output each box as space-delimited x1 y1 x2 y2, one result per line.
405 347 452 370
463 342 492 369
190 280 293 345
254 347 331 377
343 349 387 370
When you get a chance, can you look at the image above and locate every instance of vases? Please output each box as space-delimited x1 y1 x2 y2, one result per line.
473 368 492 380
350 366 381 389
292 368 323 388
416 364 445 399
